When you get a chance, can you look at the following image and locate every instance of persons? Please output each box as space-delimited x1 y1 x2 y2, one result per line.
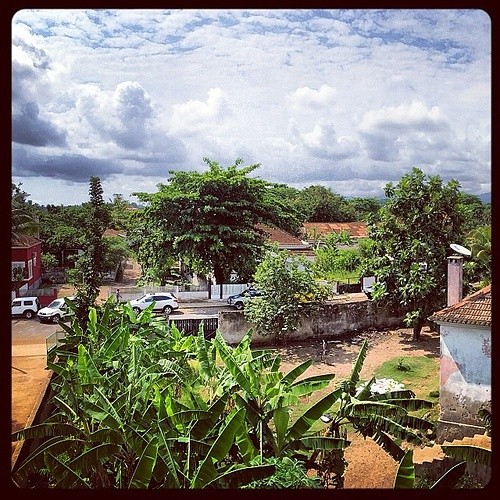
116 289 123 304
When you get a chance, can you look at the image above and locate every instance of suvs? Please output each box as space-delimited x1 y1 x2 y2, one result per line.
228 285 265 310
37 298 78 323
127 293 180 314
11 297 38 318
